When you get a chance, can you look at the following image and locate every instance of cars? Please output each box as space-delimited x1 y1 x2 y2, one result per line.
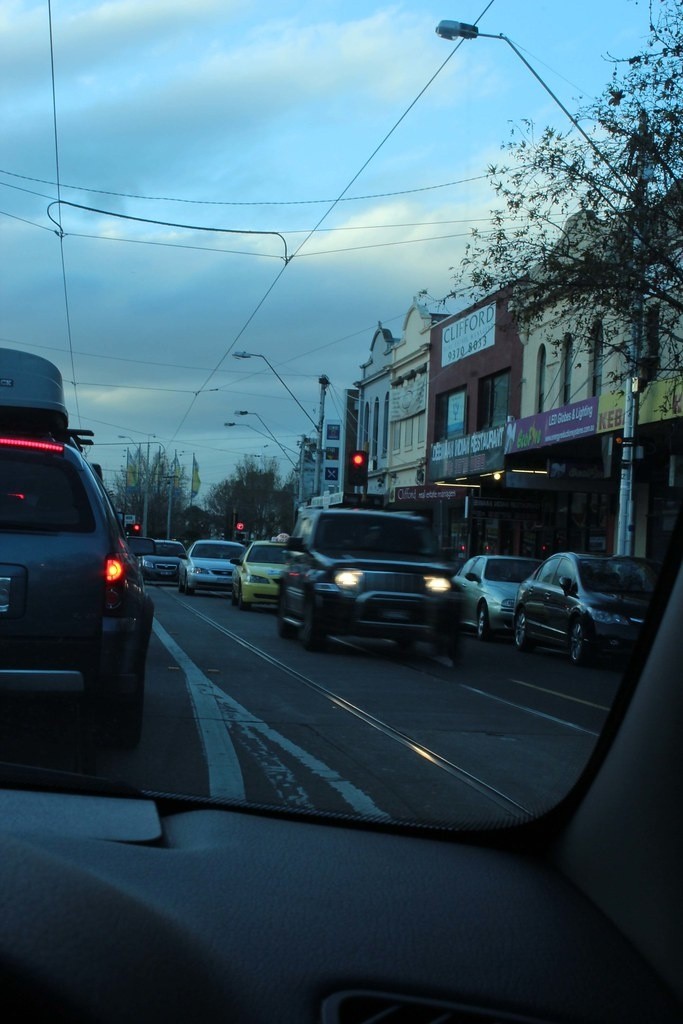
452 553 540 634
177 540 244 594
515 550 665 670
136 539 185 584
230 530 287 605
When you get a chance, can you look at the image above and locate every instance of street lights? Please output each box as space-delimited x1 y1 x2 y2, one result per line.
436 21 650 558
234 410 305 509
233 350 333 500
120 434 150 541
221 421 308 511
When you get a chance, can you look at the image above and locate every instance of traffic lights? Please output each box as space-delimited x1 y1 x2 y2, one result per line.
348 451 367 486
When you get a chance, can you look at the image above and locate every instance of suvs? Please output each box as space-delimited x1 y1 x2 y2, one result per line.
0 347 155 732
281 506 473 672
